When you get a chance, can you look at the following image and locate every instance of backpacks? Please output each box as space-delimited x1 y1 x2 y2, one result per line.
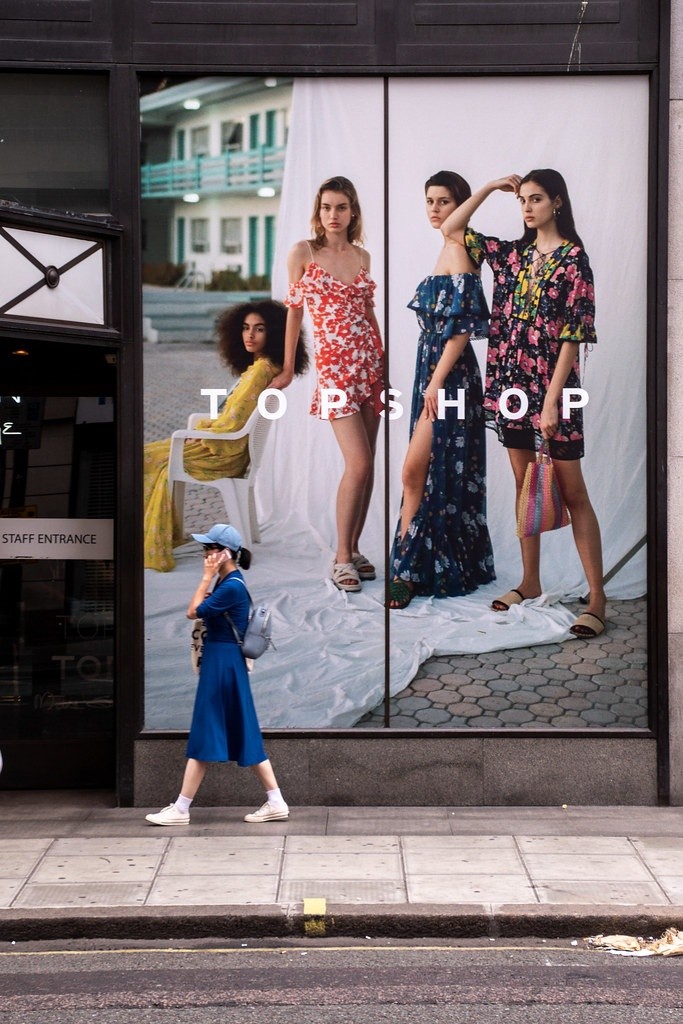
218 577 277 660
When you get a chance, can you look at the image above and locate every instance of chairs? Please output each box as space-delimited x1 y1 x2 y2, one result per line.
165 390 279 549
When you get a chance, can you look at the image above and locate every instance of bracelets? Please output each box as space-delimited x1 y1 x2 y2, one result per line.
202 577 211 581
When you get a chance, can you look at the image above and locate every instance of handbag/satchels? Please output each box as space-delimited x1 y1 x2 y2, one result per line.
516 442 571 540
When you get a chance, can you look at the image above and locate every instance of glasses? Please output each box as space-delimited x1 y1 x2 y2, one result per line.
201 545 221 551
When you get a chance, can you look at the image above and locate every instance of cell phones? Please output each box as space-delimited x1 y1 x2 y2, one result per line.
216 548 232 564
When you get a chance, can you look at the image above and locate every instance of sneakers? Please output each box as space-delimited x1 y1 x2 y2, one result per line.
145 802 190 827
244 801 289 823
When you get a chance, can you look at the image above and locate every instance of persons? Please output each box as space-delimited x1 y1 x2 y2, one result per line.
265 176 386 591
439 169 607 637
145 523 288 826
387 171 496 609
141 300 307 574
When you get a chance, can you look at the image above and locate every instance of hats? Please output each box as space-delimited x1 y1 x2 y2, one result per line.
190 524 242 552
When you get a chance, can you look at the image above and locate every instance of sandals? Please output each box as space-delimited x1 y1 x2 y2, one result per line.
331 559 361 592
352 553 376 579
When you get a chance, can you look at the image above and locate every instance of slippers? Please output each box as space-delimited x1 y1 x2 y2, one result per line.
491 589 524 611
569 612 605 637
384 577 417 609
